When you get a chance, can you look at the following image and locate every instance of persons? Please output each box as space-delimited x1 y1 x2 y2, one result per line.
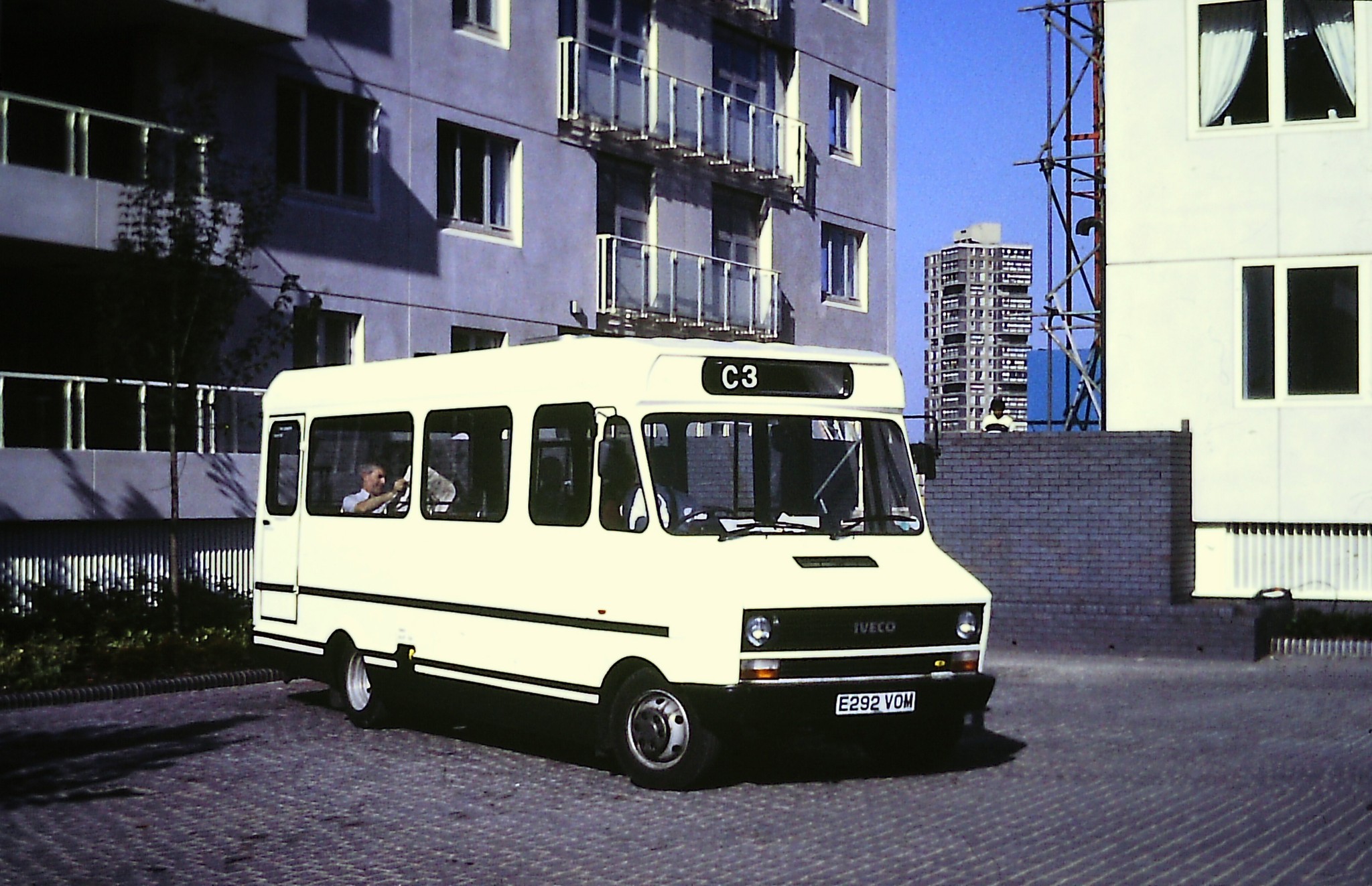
340 461 406 515
533 455 578 521
979 398 1016 433
620 445 709 534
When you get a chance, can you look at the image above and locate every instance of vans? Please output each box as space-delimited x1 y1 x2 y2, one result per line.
246 296 997 791
